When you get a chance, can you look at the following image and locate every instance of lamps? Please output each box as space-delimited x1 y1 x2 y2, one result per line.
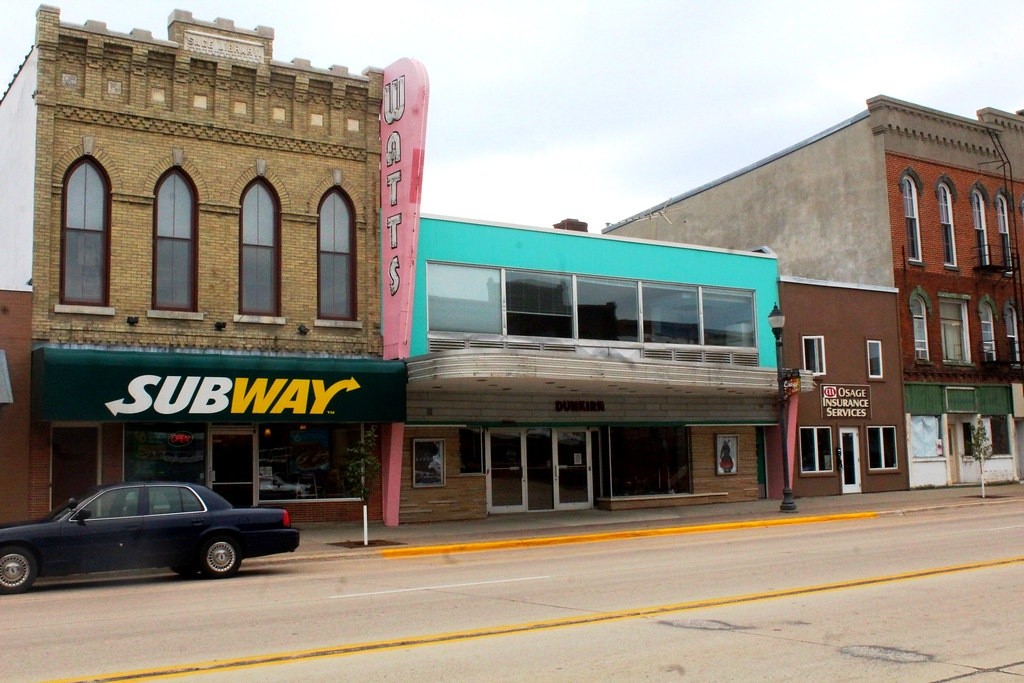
297 324 310 335
215 322 226 331
126 317 139 326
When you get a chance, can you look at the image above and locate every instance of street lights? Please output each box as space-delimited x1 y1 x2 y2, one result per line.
769 302 798 514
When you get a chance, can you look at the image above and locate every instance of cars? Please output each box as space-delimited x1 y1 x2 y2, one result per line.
0 479 302 596
